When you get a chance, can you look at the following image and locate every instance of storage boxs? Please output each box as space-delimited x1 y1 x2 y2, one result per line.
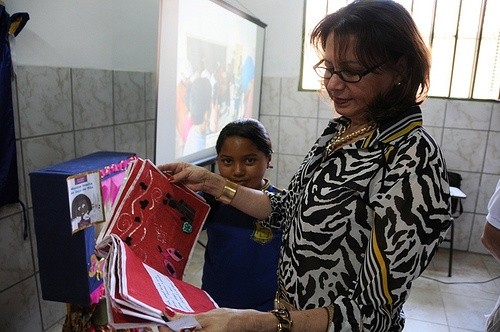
27 150 141 307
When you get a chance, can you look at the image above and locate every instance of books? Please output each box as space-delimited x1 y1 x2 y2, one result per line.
93 158 220 330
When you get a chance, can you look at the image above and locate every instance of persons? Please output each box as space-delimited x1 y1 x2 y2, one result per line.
194 119 283 313
176 43 255 157
157 0 452 332
481 179 500 332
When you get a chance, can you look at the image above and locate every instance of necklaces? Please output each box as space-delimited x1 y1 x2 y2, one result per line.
323 124 377 161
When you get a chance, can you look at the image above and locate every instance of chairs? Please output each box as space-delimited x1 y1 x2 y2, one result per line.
442 171 466 277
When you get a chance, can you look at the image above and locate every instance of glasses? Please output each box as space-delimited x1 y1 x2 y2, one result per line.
312 58 389 83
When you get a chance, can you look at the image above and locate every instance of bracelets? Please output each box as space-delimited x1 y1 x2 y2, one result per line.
271 307 293 332
215 178 237 205
